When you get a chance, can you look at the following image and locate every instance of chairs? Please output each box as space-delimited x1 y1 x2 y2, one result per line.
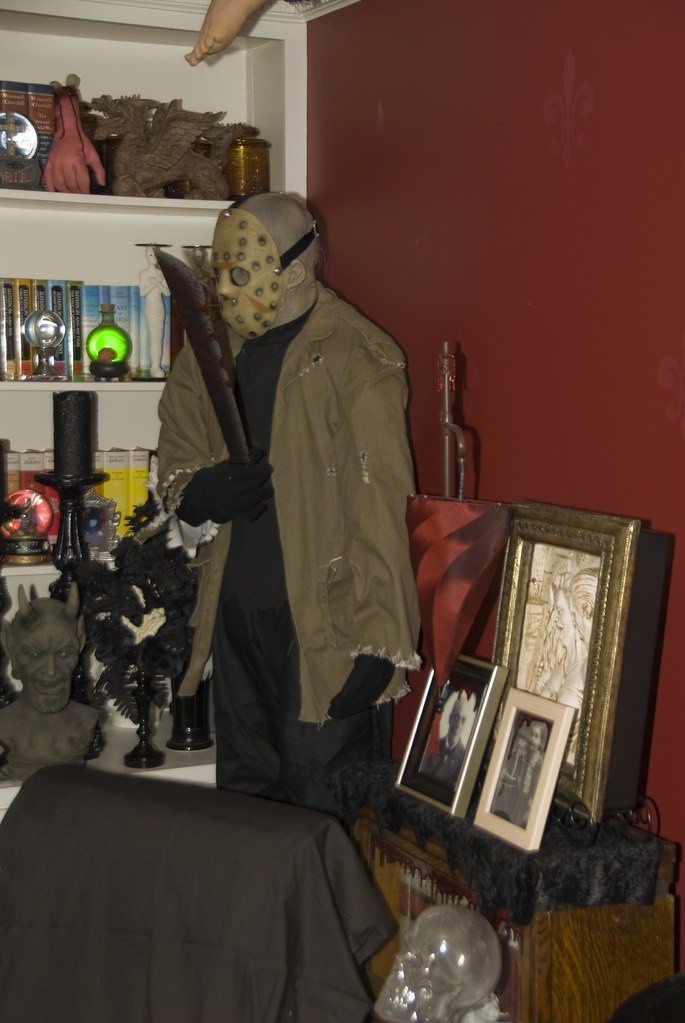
1 762 397 1022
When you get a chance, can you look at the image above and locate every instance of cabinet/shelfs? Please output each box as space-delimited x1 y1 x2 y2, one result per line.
353 794 676 1023
0 1 306 825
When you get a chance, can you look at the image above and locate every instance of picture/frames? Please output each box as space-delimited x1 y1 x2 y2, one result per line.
393 651 510 817
486 497 643 823
472 685 576 853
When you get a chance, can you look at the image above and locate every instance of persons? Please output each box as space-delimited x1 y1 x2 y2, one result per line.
156 579 194 661
422 698 468 789
521 723 546 824
0 582 100 775
185 0 266 66
20 499 37 529
42 86 106 194
158 196 421 824
192 248 216 310
140 248 170 378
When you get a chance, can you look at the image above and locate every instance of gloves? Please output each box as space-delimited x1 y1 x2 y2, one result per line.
327 652 396 720
174 448 274 528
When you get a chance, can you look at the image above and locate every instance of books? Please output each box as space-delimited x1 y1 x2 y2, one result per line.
0 277 185 378
3 448 158 552
